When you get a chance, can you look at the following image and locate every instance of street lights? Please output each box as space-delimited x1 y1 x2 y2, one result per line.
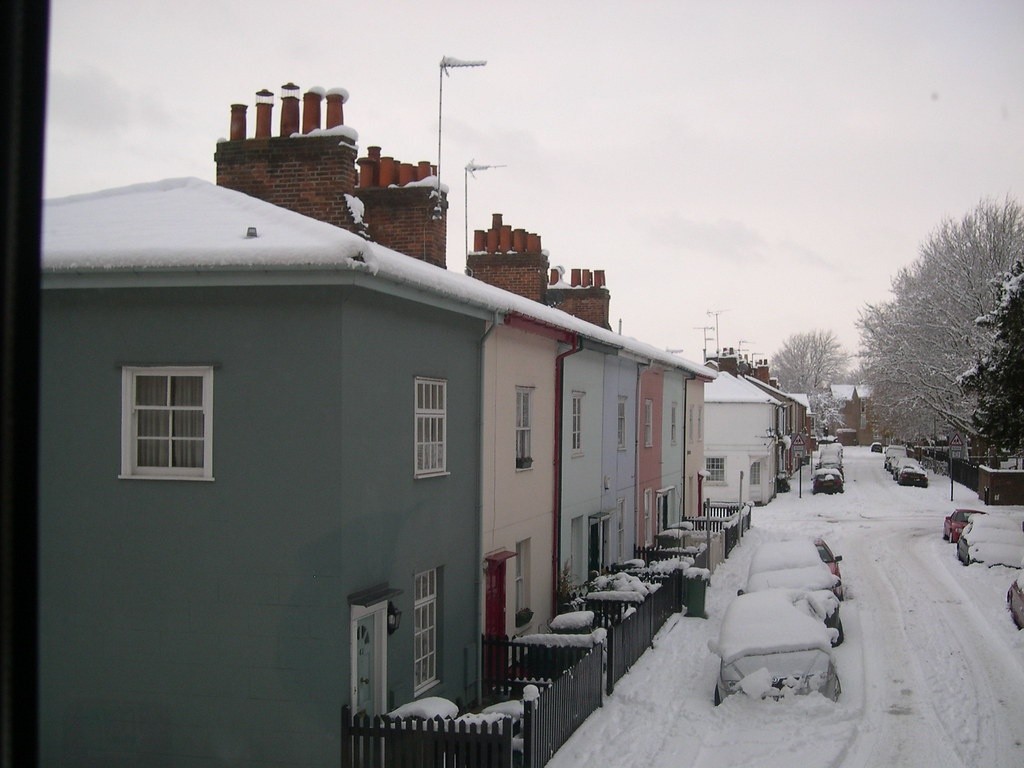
462 162 508 263
438 55 488 201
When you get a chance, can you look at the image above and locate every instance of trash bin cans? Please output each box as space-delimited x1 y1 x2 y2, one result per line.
683 567 710 617
804 455 809 465
778 471 786 491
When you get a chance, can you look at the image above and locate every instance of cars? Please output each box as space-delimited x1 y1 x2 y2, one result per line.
871 442 883 454
885 446 929 488
955 518 1023 571
748 536 846 601
813 442 848 494
1007 576 1024 628
943 508 989 543
712 587 844 709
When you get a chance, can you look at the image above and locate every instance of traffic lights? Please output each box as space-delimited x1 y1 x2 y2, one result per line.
825 426 829 437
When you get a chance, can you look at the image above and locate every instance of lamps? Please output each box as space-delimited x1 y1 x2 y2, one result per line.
387 601 401 634
